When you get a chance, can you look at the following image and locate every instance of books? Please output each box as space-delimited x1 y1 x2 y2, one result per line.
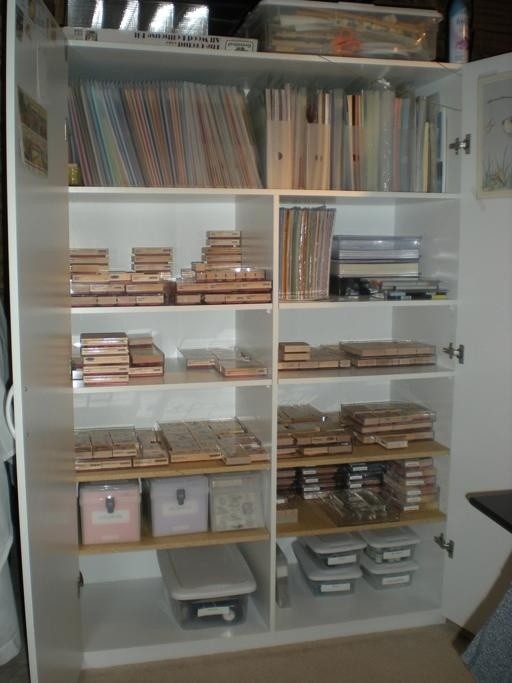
67 77 447 192
330 235 438 296
279 206 336 300
156 543 258 630
235 0 443 61
291 525 422 597
75 471 266 546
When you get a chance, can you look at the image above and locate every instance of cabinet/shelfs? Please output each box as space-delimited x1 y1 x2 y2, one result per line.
448 0 471 62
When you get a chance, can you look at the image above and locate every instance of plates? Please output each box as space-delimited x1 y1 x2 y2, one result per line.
5 0 512 683
